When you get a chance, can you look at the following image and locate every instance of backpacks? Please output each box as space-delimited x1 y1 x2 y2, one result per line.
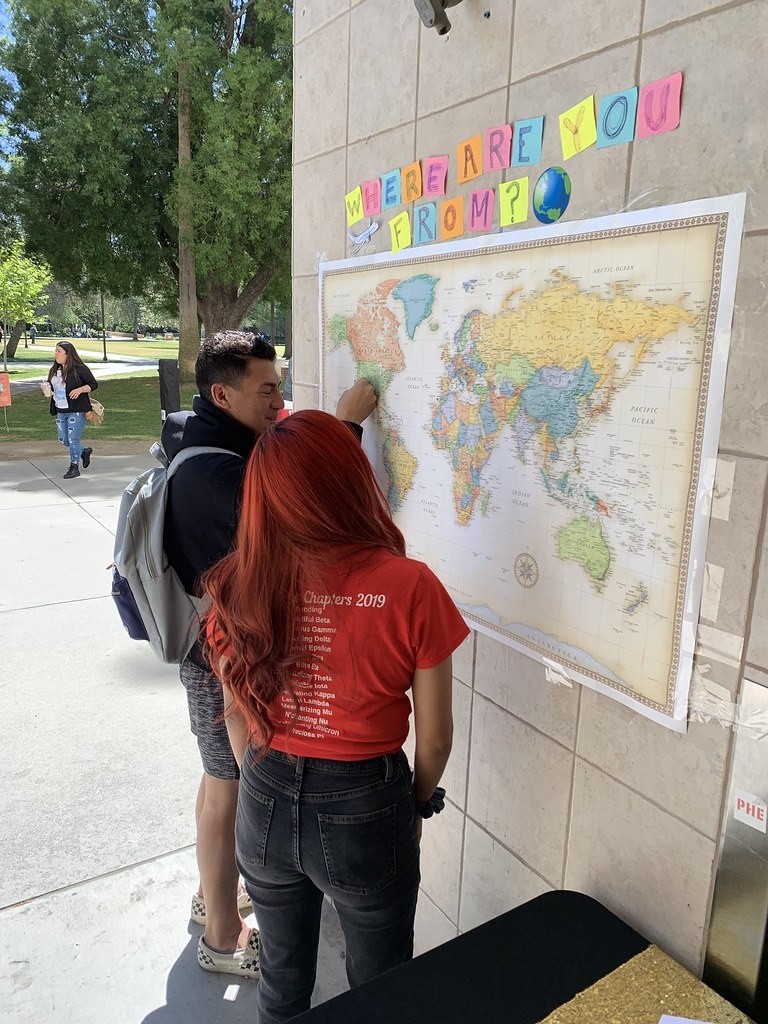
106 442 245 664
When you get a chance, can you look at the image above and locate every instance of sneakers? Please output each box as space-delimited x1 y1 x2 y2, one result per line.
63 463 80 479
81 448 92 468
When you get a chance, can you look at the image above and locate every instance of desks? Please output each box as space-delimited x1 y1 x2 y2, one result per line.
281 888 759 1024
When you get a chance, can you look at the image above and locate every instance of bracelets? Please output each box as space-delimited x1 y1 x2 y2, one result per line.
420 787 446 819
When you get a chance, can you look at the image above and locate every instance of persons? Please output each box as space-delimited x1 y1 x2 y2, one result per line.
206 409 471 1024
179 329 377 977
0 326 3 342
87 327 91 338
30 324 37 343
40 341 98 478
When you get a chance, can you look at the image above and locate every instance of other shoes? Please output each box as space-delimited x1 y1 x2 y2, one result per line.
191 873 253 926
197 928 261 979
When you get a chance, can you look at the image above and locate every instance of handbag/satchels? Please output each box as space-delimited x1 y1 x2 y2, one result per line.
86 397 105 426
